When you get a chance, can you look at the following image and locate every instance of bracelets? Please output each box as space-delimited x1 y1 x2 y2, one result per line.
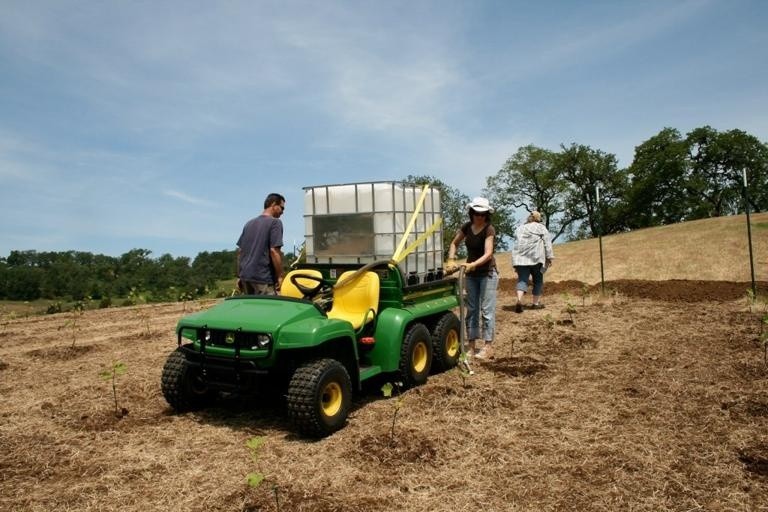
237 275 241 279
474 261 480 266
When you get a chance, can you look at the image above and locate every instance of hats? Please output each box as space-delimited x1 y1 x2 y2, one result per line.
465 198 495 214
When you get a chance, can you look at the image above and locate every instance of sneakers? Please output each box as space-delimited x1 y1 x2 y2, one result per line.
475 346 494 359
516 301 523 312
465 345 475 363
532 302 544 309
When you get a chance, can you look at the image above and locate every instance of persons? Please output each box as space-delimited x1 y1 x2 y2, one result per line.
236 193 286 297
449 198 499 360
512 210 555 313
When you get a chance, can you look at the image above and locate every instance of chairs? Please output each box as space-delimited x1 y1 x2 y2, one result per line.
278 268 324 301
325 270 381 335
